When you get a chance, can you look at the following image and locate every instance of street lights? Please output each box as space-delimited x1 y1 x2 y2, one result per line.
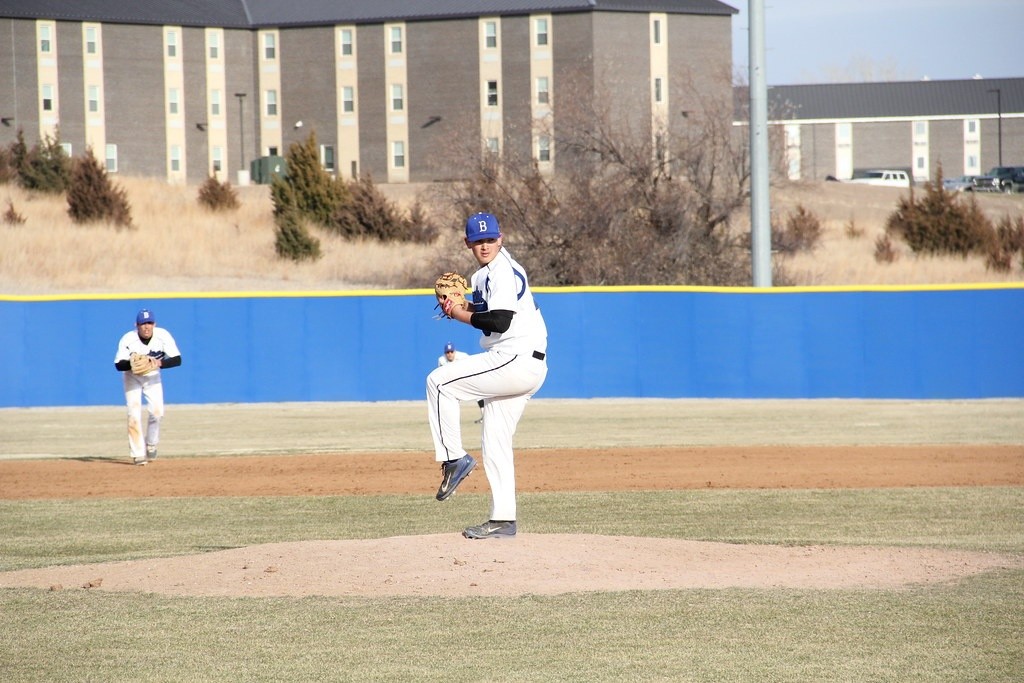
988 89 1002 167
233 92 247 169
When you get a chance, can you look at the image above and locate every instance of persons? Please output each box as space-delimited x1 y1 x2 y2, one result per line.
438 343 484 423
114 309 180 464
426 212 547 538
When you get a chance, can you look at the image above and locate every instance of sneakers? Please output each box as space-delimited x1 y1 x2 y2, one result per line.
462 521 517 539
134 455 148 466
436 454 477 501
146 443 158 460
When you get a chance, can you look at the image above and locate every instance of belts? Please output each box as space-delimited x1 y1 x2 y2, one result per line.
533 351 544 360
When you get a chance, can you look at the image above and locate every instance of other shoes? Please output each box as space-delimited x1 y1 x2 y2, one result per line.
475 417 483 424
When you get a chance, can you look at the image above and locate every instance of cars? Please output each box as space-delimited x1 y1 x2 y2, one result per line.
943 174 975 193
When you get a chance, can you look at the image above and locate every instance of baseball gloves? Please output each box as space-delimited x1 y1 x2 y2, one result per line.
435 271 468 320
129 353 157 375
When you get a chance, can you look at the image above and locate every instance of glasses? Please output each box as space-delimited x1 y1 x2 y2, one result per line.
446 351 453 354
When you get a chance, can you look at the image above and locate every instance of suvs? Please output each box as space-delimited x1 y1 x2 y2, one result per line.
849 169 910 187
970 166 1024 195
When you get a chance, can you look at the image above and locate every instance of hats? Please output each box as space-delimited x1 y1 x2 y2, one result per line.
137 309 155 325
466 212 500 242
444 342 455 353
